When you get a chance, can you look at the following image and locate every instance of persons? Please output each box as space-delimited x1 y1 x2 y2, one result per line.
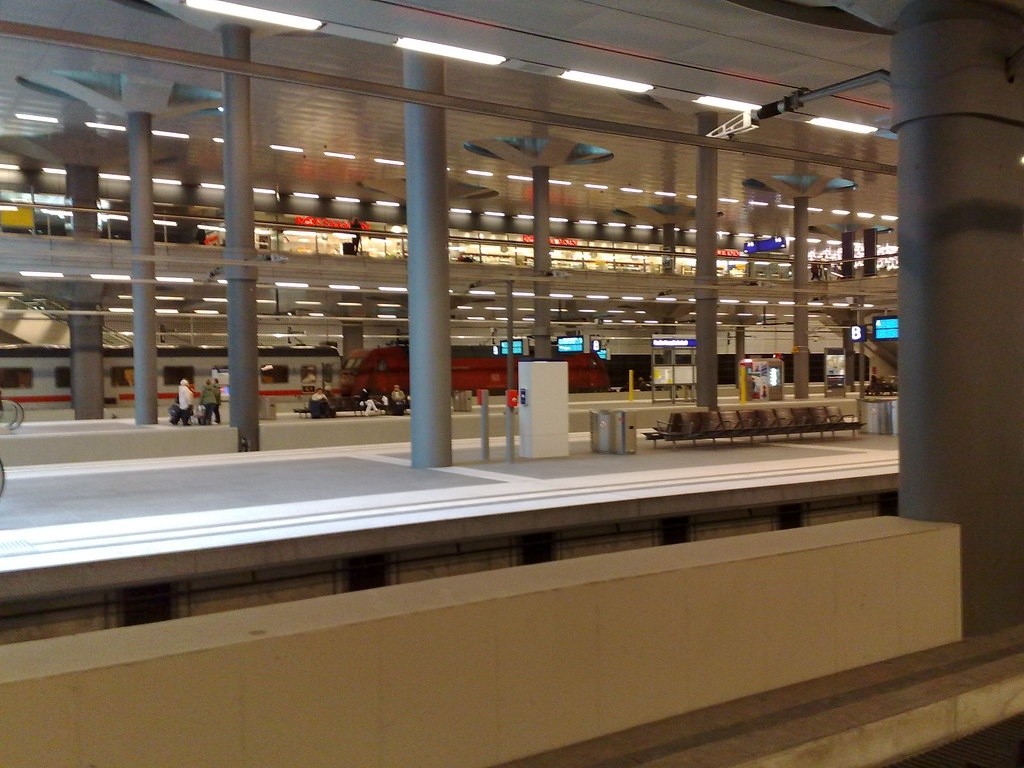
359 388 381 416
391 385 406 416
865 375 878 396
638 377 646 391
167 378 221 426
311 388 329 419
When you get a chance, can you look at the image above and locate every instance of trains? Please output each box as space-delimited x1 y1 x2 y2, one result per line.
0 344 343 410
340 345 611 397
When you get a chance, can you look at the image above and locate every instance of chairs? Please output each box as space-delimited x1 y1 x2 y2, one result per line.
294 396 410 420
865 383 898 395
641 406 868 448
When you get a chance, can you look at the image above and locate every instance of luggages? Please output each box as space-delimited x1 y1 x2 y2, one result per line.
167 403 182 424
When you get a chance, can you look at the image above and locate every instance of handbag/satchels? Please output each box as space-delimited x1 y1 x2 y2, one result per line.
195 404 206 418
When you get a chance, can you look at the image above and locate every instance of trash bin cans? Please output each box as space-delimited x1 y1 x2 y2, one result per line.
454 390 473 412
259 395 277 421
856 398 899 435
589 409 638 455
